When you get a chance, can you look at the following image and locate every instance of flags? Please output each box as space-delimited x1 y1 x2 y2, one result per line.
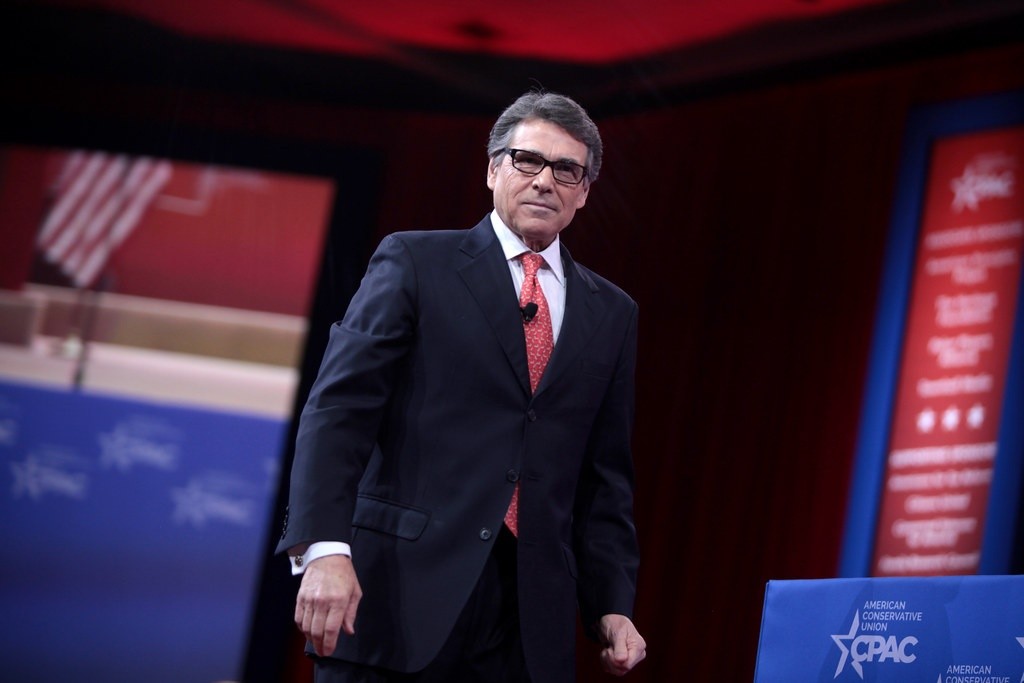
34 151 177 287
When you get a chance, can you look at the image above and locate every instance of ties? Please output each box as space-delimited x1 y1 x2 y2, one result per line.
503 253 554 539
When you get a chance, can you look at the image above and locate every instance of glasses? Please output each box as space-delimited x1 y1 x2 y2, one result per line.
492 147 591 185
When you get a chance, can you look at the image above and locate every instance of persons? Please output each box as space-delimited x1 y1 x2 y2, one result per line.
276 93 645 683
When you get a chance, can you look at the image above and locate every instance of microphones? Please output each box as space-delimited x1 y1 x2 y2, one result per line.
522 302 538 321
